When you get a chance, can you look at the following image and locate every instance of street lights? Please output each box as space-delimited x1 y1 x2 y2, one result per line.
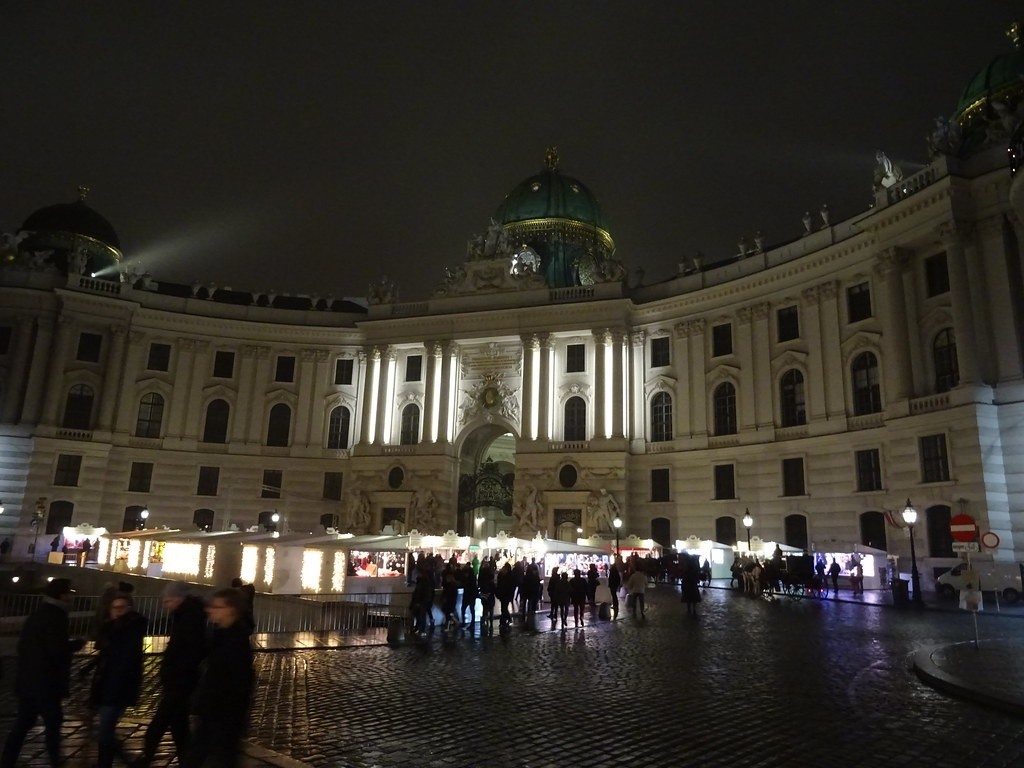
27 495 48 562
742 507 756 551
902 497 924 607
139 504 150 529
612 512 622 557
271 508 280 531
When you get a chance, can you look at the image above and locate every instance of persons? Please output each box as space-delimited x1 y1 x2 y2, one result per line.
83 540 91 553
593 488 620 533
347 490 369 528
519 484 537 528
772 544 782 565
349 543 712 637
80 578 258 768
815 559 826 583
482 217 502 256
0 577 85 768
50 538 59 552
62 546 68 558
414 489 435 525
828 557 841 592
850 564 864 595
0 538 9 554
730 557 740 587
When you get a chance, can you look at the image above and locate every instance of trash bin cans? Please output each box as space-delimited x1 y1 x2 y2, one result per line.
891 578 910 602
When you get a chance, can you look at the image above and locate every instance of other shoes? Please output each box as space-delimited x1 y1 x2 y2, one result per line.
641 611 645 617
563 619 567 626
580 618 584 627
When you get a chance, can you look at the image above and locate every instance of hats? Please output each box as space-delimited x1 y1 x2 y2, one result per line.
160 582 189 596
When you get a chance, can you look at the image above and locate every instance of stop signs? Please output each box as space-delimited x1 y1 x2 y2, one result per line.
948 514 977 542
